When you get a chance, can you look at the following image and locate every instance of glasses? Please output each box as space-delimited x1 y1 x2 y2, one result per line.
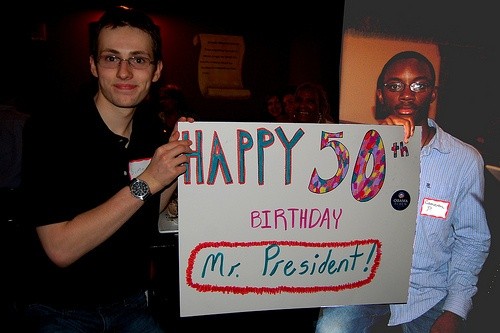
96 54 156 70
383 78 434 93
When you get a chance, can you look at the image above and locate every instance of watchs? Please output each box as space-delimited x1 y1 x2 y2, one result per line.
127 178 152 201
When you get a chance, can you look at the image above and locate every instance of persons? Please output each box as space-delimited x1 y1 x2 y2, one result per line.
2 81 334 189
9 7 206 333
315 51 491 333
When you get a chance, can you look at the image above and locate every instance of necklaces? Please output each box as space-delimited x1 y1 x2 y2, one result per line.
316 112 323 123
419 126 431 151
